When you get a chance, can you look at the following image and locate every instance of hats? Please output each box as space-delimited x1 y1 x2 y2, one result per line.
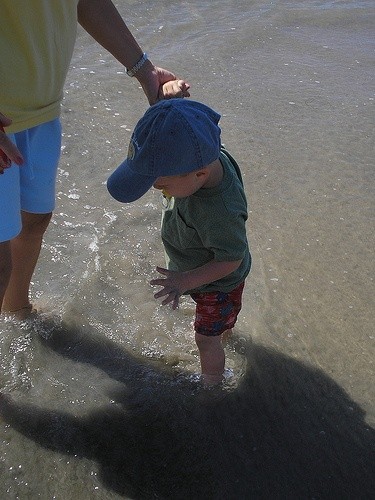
107 99 221 203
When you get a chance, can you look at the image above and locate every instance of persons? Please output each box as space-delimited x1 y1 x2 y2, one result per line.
107 79 252 388
0 0 190 320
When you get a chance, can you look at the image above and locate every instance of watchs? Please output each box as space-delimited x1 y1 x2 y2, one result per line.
126 51 148 77
